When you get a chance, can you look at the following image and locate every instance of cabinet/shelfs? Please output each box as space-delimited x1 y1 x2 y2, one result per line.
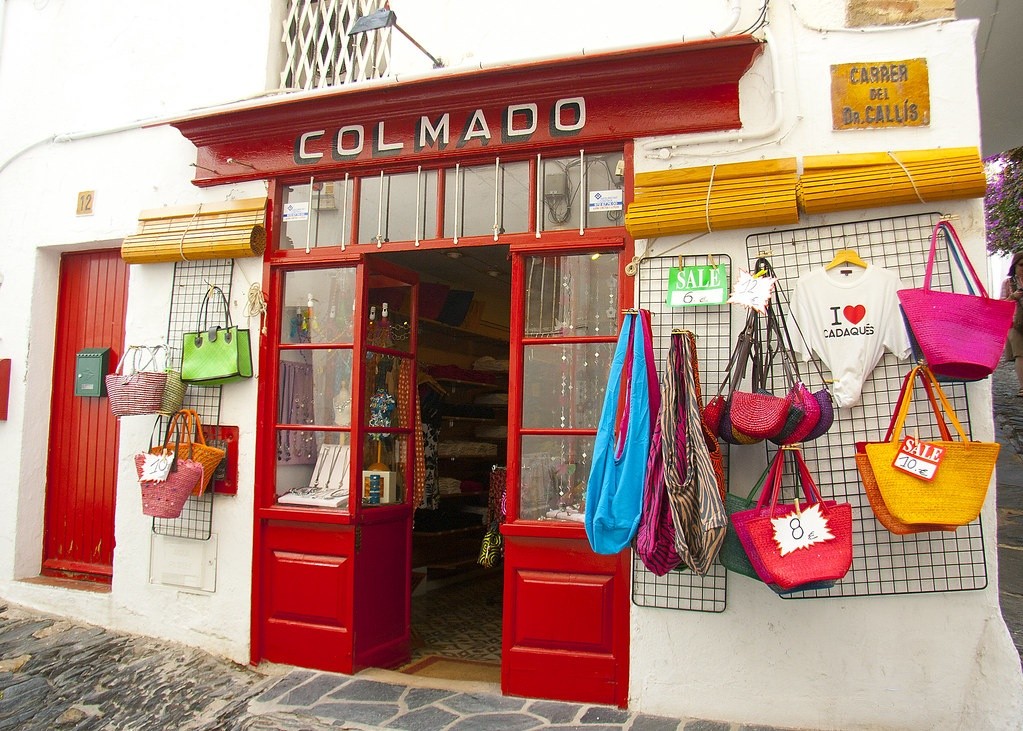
364 304 509 570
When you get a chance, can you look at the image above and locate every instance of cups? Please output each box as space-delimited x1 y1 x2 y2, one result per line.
369 474 381 505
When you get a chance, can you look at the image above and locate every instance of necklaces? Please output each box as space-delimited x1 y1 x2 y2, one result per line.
277 361 326 460
314 445 350 489
333 396 351 413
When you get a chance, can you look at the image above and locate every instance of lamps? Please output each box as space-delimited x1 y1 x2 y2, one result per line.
349 11 442 67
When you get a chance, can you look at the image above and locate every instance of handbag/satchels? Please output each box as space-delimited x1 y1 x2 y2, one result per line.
133 407 225 518
476 529 502 569
896 219 1014 385
857 365 1003 535
103 343 184 416
702 258 834 444
716 448 853 596
583 310 727 575
181 286 253 387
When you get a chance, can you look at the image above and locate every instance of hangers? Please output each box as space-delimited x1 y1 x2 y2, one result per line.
826 235 867 269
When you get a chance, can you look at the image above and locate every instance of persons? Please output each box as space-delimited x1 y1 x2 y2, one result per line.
999 251 1023 397
